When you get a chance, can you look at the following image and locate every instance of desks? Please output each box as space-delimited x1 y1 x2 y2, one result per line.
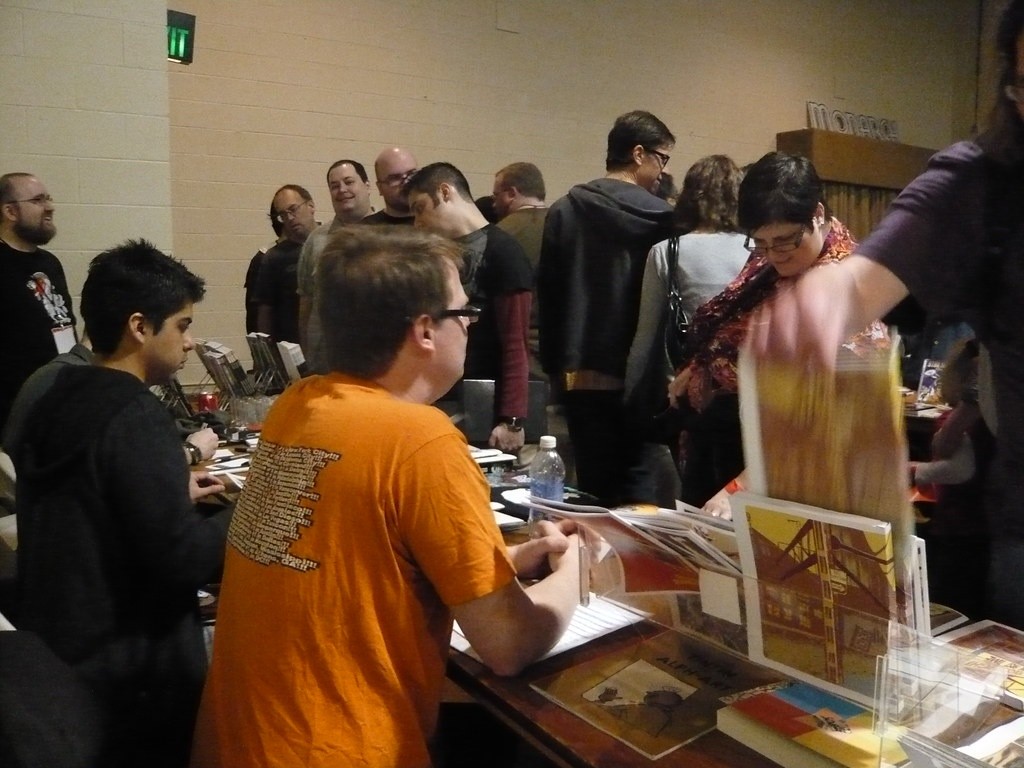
190 443 1024 768
903 391 949 462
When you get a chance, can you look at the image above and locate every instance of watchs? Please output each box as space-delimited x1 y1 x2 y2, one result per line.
499 416 526 429
181 442 203 465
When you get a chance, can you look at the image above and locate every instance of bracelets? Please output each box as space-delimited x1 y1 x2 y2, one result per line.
724 479 744 494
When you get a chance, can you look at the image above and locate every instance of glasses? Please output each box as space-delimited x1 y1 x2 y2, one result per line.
743 222 806 253
405 305 481 323
277 200 309 223
8 195 53 206
379 172 418 187
643 146 671 167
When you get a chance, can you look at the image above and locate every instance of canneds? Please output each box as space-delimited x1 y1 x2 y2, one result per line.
198 391 219 412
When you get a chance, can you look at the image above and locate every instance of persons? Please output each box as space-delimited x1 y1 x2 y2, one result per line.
246 0 1024 622
194 225 591 768
0 175 226 768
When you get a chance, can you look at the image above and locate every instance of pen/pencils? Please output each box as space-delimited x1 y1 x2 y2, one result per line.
208 466 250 476
234 447 256 454
215 453 251 462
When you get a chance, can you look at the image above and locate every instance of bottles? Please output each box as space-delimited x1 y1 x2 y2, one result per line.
527 436 566 523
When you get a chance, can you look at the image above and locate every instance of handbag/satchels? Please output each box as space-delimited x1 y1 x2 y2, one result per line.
623 234 690 441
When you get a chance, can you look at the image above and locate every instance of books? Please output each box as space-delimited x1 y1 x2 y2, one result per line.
521 337 1024 768
